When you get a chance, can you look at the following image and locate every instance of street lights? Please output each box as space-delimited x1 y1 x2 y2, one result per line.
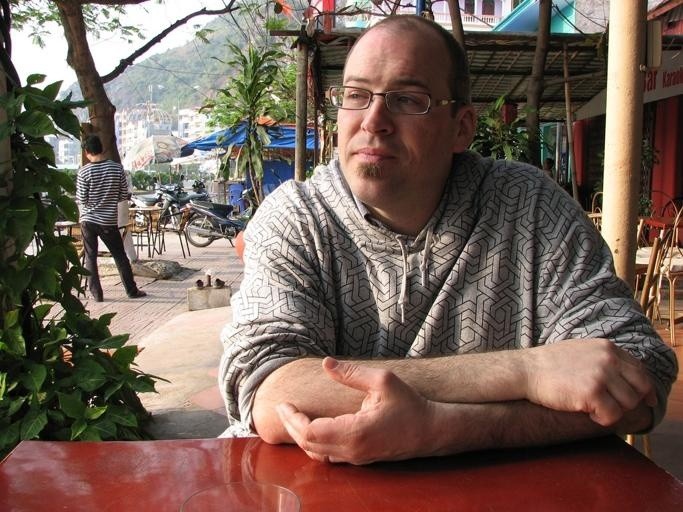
148 83 164 135
176 85 200 138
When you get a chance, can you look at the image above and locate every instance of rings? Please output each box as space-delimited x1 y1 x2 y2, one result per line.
321 453 329 464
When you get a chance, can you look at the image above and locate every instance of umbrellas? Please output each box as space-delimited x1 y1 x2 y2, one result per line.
121 134 194 187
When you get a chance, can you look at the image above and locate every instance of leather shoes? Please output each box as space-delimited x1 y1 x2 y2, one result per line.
127 290 145 298
94 297 103 302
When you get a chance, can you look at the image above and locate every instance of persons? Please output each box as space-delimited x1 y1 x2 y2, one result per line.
585 159 602 211
74 134 145 302
212 13 678 467
542 158 554 177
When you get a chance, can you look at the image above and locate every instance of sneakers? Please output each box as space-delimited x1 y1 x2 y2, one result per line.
212 279 224 289
192 279 203 289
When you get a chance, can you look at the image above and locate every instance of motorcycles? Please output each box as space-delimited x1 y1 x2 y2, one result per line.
128 179 257 248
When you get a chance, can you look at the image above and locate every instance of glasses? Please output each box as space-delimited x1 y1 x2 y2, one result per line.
324 85 456 116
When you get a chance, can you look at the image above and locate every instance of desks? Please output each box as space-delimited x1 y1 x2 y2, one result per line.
0 437 683 512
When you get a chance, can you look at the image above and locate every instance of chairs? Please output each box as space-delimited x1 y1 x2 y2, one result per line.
587 191 683 348
54 201 191 298
